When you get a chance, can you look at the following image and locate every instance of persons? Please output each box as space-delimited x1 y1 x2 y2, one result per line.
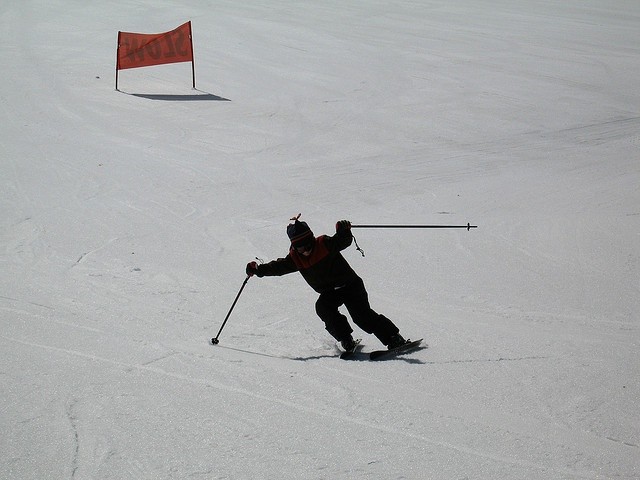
246 222 405 352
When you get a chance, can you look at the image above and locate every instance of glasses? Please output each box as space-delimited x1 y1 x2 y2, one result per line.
296 241 313 253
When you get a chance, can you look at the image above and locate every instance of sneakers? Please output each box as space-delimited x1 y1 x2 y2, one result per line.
341 335 354 351
387 334 406 350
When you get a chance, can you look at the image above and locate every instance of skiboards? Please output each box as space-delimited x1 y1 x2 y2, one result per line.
341 338 423 360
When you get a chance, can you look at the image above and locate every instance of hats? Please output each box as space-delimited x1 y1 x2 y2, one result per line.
286 213 313 247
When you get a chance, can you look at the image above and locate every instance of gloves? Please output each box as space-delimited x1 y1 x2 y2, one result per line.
246 262 258 277
336 220 352 232
